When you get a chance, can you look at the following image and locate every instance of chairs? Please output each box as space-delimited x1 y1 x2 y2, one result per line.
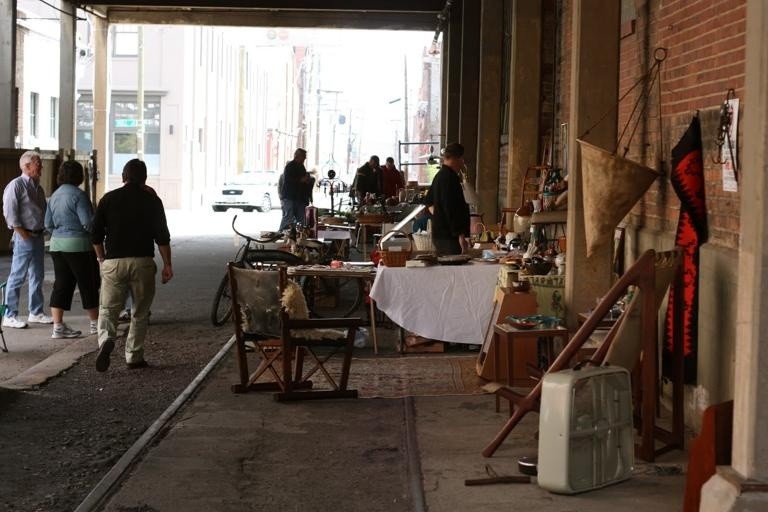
227 261 363 404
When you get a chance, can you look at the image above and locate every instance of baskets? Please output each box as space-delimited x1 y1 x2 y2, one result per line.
412 219 437 252
526 258 552 275
379 230 412 267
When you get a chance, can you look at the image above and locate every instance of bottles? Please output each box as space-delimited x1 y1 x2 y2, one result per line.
542 192 555 212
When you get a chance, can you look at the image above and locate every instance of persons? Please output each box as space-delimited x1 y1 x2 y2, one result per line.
0 150 54 329
42 161 105 339
85 158 173 373
116 181 158 322
283 147 315 227
277 160 298 231
379 157 403 197
426 142 472 258
355 155 384 202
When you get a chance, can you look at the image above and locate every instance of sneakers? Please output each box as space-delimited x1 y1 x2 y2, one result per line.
89 322 99 334
28 313 53 324
52 325 80 337
129 361 146 367
96 340 114 372
2 317 28 328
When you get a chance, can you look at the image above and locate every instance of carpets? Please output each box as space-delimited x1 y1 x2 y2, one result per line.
254 354 491 399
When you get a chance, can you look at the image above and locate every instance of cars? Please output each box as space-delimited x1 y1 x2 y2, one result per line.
317 178 347 195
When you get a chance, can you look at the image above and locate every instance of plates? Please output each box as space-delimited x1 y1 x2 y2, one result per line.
434 254 472 265
509 322 536 330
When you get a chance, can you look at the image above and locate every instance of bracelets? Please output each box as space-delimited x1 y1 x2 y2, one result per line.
96 257 105 262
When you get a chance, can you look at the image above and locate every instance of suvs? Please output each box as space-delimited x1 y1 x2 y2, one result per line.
210 168 282 214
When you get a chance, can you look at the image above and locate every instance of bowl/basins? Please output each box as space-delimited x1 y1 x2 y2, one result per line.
513 281 530 291
526 261 552 275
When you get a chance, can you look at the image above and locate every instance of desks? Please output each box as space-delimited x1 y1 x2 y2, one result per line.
287 265 380 356
494 324 568 418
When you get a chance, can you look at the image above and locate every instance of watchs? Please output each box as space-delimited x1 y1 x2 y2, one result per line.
17 230 26 236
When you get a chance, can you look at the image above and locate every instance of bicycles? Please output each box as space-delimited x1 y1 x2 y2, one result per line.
208 214 367 331
